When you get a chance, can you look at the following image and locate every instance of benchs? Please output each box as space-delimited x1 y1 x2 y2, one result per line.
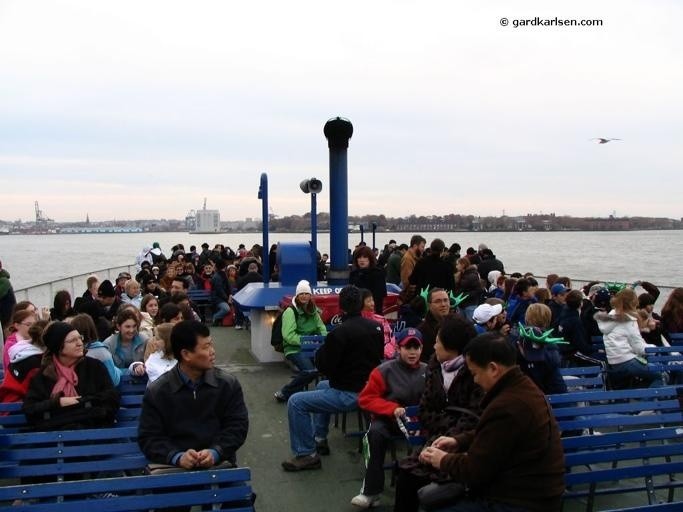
300 295 682 510
1 254 252 510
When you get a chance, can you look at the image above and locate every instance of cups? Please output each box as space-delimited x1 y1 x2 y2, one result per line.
66 336 83 343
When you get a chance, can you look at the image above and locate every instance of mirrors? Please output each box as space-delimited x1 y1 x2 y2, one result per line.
592 136 622 144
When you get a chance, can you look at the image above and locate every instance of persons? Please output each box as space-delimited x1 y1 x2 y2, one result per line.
421 333 562 510
279 286 386 473
394 315 488 511
319 232 682 436
21 321 120 506
135 320 250 496
135 243 276 330
350 327 435 507
2 269 205 402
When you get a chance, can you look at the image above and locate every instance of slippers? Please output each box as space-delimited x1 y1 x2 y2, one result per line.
271 305 297 351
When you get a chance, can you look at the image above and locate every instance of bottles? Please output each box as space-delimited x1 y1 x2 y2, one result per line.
472 304 502 324
551 284 571 294
397 328 423 345
296 280 311 296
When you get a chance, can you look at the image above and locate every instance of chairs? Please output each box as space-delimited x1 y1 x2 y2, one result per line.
315 436 329 455
275 391 287 401
281 455 321 470
351 494 380 507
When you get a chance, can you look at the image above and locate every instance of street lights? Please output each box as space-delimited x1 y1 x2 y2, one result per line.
146 460 231 475
